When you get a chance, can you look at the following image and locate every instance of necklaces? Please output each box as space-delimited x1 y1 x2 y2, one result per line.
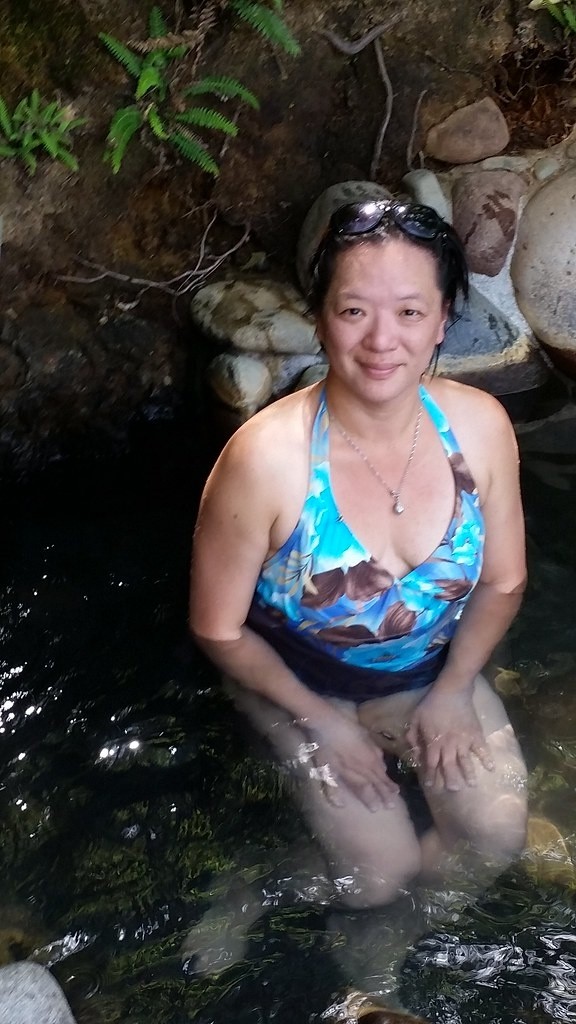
328 383 424 514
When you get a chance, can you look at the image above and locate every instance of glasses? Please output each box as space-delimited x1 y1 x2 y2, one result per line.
317 199 469 301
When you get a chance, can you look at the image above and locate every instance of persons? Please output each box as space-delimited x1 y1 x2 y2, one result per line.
178 201 528 1012
